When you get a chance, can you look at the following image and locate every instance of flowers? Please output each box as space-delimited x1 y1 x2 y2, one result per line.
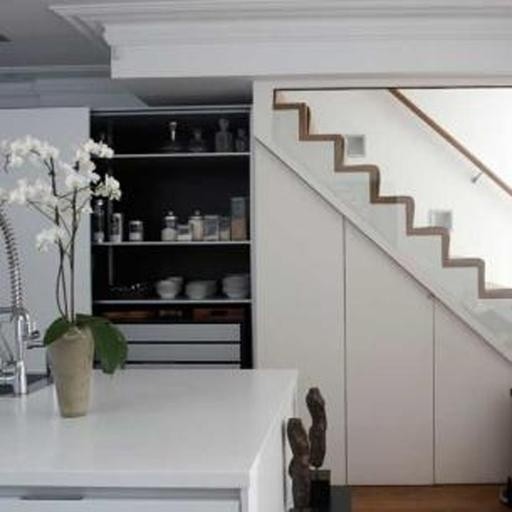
0 133 132 379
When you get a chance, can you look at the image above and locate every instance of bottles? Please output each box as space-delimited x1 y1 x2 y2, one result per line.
159 117 249 153
161 195 249 241
92 199 144 243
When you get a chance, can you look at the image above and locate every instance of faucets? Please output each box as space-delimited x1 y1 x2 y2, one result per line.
0 304 40 393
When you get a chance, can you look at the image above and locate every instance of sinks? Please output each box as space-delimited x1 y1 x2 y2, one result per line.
0 368 53 398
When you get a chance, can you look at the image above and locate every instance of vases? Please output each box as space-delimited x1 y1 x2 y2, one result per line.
44 323 96 417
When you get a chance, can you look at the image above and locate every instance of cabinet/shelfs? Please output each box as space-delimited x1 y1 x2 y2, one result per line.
87 104 253 303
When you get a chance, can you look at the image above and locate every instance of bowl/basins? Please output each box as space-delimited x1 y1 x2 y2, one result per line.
155 276 250 299
